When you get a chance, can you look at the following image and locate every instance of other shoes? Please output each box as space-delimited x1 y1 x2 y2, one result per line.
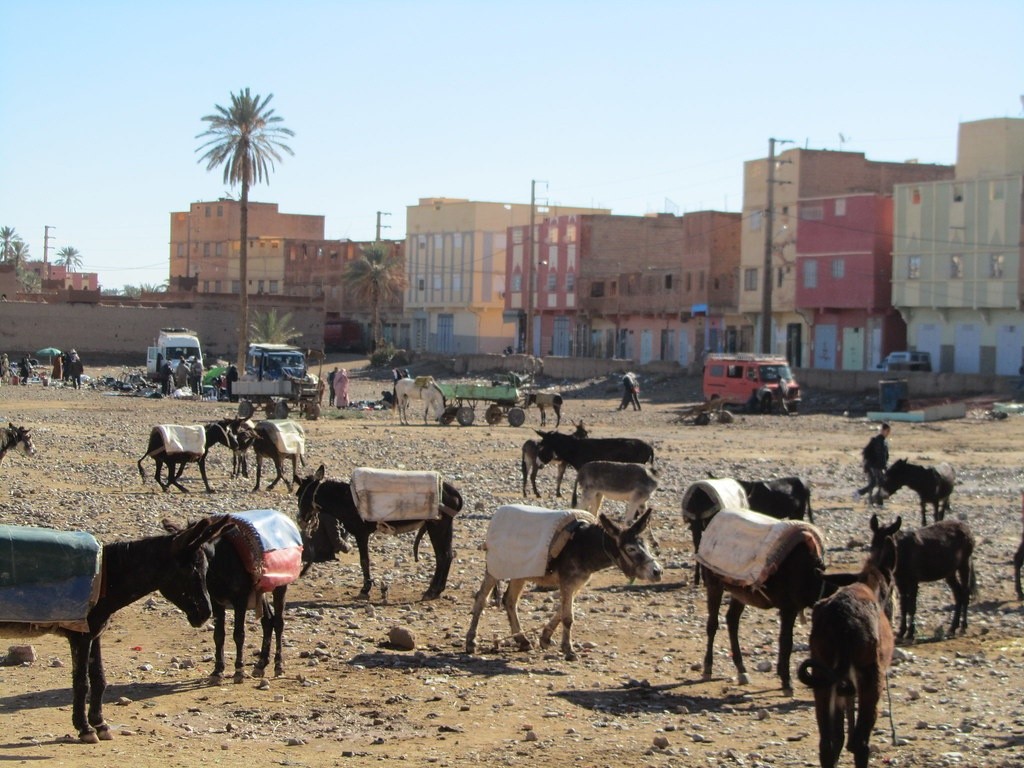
877 504 888 511
854 492 861 503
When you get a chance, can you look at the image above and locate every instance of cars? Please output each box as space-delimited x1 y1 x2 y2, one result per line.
244 347 324 404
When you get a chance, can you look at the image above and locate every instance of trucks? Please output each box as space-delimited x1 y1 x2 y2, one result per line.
146 328 207 380
875 351 932 372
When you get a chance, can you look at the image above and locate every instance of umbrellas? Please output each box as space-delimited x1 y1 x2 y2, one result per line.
203 368 225 384
36 348 61 375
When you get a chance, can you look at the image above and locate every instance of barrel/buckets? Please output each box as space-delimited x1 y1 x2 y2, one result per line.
43 379 49 386
13 375 19 385
879 378 910 414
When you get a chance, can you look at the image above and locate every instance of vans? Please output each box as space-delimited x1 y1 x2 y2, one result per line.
703 352 801 411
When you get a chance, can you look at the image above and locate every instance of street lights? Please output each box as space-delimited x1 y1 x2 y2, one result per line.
761 226 787 353
527 259 547 355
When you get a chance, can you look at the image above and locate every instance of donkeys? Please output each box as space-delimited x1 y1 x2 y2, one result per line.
396 379 458 426
0 415 1024 768
524 393 563 429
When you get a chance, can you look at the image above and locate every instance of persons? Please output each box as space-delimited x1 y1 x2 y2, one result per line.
52 349 83 389
758 375 789 415
205 356 210 370
328 367 349 409
852 423 890 509
0 353 10 383
21 353 34 385
160 356 203 395
616 369 641 410
211 362 239 400
382 368 410 405
